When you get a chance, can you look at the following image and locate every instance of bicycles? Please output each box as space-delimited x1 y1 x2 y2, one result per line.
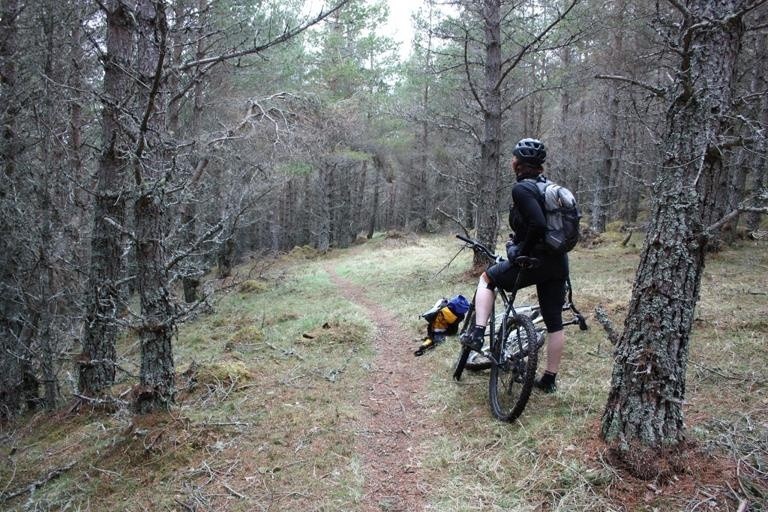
460 270 588 374
451 231 541 427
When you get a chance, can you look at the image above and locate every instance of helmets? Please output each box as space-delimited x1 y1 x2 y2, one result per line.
511 138 546 165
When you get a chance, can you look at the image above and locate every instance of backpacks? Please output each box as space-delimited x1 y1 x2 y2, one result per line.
512 173 583 257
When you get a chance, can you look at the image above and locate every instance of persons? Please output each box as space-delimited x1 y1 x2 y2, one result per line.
460 137 581 395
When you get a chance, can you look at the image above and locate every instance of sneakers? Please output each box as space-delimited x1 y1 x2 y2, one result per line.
460 333 484 351
533 378 557 393
